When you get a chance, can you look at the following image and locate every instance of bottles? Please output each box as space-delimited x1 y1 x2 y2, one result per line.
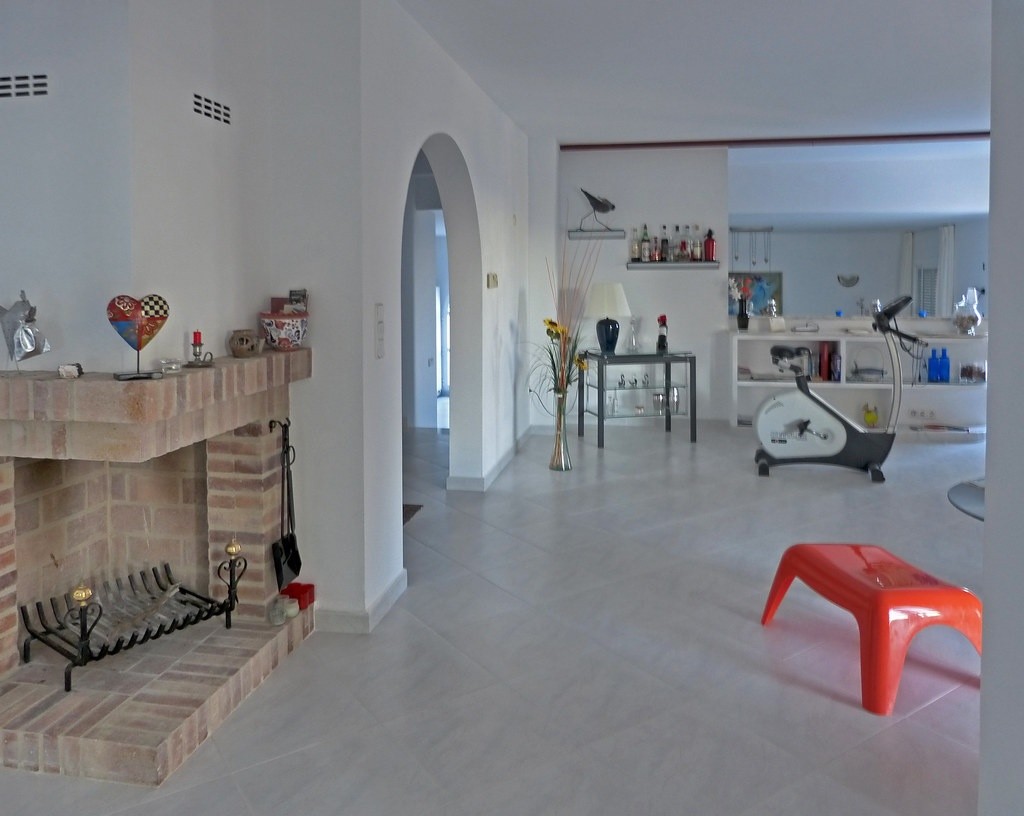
607 394 613 416
691 224 703 262
939 347 950 382
630 228 641 263
613 389 619 416
656 315 668 354
650 234 661 263
927 348 938 382
681 225 691 261
661 226 673 262
704 229 716 261
673 226 681 261
641 223 650 263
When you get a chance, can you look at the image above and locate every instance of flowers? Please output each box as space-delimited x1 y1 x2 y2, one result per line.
523 195 610 469
728 273 754 301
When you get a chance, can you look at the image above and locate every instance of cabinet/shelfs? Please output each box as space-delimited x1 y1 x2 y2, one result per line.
731 333 987 431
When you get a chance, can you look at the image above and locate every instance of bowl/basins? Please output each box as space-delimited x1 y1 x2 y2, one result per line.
259 311 309 352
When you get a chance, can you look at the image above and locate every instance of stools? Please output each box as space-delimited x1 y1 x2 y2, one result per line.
758 542 982 717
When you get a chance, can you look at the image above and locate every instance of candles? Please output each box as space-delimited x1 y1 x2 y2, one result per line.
193 329 202 345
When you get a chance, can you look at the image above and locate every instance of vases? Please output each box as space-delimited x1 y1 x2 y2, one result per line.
550 393 572 472
738 298 750 331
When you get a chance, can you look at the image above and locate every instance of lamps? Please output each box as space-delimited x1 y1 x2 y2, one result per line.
584 282 633 356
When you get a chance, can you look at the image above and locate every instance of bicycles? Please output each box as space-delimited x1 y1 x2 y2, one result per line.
754 297 927 481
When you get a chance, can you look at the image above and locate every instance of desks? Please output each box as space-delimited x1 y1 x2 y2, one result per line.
577 348 698 449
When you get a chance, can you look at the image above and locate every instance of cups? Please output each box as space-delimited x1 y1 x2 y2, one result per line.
669 386 679 413
653 393 666 415
635 406 645 416
